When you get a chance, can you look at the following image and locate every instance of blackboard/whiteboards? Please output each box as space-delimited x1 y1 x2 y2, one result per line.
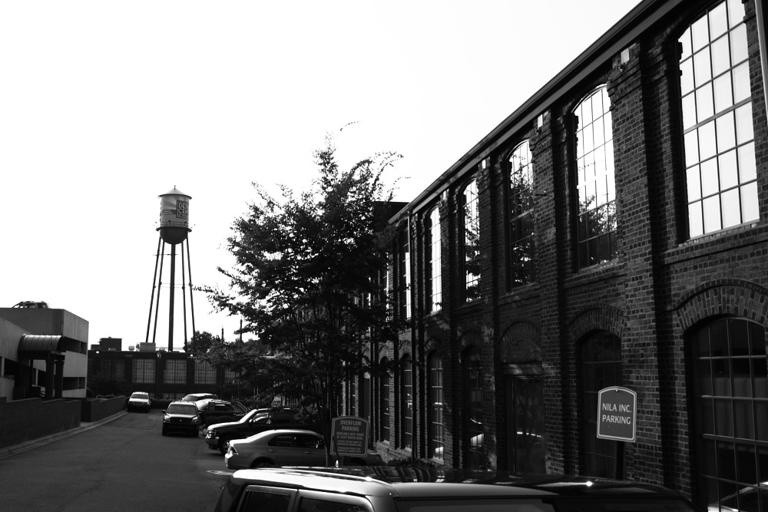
329 416 370 458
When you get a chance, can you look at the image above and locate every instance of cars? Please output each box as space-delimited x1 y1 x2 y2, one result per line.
224 429 327 470
181 393 236 424
206 406 299 455
161 400 201 437
127 391 152 413
